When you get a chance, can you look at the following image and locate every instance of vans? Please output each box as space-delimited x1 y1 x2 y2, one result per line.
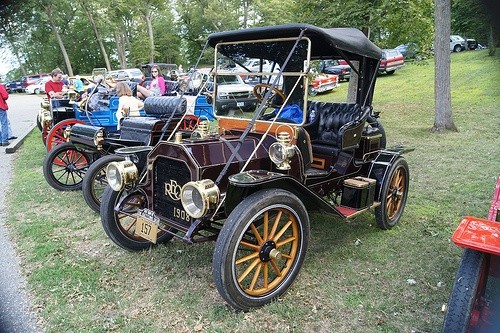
23 73 50 90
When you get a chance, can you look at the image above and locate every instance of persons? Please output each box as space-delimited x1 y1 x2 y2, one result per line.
73 75 83 93
114 82 145 131
106 65 165 99
46 68 67 104
0 78 18 146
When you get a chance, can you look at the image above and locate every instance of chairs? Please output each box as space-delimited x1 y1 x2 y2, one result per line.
258 99 370 170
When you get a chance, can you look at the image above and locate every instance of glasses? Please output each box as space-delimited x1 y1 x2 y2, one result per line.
152 71 158 73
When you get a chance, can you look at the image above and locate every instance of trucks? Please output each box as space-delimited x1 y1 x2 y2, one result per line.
92 68 106 78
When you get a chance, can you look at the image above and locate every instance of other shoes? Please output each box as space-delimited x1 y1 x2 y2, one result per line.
1 143 10 146
8 136 18 140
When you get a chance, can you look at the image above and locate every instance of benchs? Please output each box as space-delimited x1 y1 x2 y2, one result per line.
121 96 187 130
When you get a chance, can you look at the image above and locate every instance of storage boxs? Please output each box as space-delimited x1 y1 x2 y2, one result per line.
340 176 377 210
312 156 336 170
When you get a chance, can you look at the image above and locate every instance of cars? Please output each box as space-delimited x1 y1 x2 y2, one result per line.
193 70 257 116
332 47 405 77
429 36 478 53
237 58 280 72
302 71 339 97
25 76 66 94
108 66 144 86
36 74 215 215
0 78 24 93
100 22 412 312
392 42 430 61
312 60 354 80
